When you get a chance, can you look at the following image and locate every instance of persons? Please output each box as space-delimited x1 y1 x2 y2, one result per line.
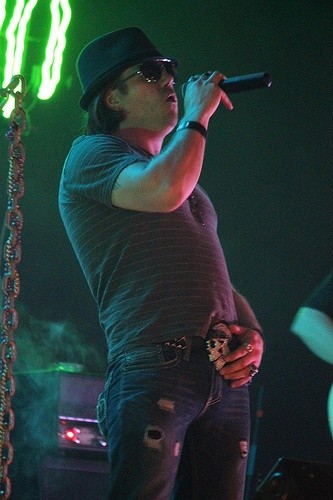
291 268 333 444
58 26 265 500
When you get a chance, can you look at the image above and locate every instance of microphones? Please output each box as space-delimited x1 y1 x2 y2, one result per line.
180 71 272 98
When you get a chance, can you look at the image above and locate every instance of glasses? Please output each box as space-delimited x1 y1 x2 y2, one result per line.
121 60 177 85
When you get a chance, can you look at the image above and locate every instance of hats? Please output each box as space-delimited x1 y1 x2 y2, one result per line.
76 26 177 111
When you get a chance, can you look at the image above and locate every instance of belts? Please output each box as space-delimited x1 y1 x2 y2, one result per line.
151 322 240 374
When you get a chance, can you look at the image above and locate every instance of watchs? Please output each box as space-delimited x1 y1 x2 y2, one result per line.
177 121 206 140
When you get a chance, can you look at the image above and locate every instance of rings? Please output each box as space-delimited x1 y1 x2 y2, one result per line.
250 365 257 376
244 342 252 352
